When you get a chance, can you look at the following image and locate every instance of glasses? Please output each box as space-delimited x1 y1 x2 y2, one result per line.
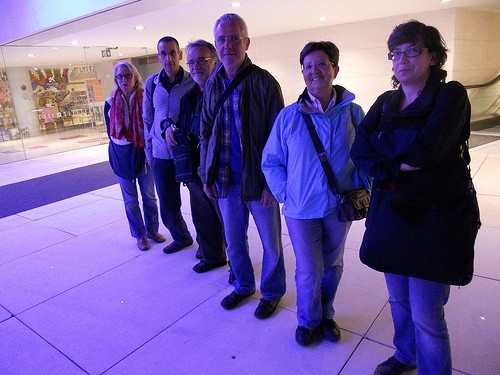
302 62 336 71
215 37 246 44
186 58 211 67
388 47 430 60
157 51 177 57
115 73 133 79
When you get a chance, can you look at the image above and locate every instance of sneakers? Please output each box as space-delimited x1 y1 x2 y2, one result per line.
221 287 255 309
295 319 340 346
254 298 279 319
373 357 417 375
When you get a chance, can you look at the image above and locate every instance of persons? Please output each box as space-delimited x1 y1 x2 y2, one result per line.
260 42 367 349
140 35 204 259
159 39 227 272
197 11 285 322
104 62 167 250
350 21 481 375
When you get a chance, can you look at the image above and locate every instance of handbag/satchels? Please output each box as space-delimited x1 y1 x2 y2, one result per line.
166 133 200 183
337 189 371 222
108 140 146 179
420 195 473 277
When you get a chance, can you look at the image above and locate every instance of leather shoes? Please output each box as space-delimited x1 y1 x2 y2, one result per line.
193 258 227 273
163 238 193 254
196 246 204 259
146 233 166 243
228 273 236 284
137 238 149 250
